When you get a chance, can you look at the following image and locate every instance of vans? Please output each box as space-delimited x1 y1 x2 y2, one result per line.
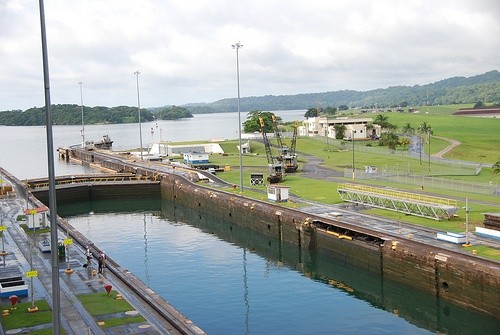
343 136 352 141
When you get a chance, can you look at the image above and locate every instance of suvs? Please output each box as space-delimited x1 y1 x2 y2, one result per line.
249 173 264 186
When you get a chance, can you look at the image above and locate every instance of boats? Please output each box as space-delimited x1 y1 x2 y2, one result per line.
69 129 114 151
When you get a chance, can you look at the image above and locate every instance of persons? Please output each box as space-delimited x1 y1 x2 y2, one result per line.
84 246 106 273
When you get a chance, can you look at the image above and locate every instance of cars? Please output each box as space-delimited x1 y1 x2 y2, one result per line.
371 134 379 140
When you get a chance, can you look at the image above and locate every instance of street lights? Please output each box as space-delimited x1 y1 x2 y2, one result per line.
231 40 244 193
134 70 144 162
78 80 85 149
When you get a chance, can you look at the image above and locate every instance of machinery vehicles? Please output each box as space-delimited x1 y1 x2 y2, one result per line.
257 115 300 184
